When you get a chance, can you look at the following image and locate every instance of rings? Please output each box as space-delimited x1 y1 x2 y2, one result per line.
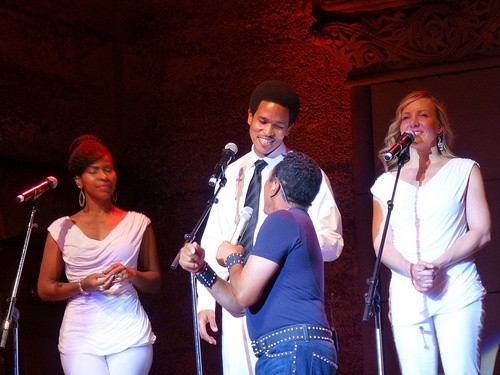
118 273 122 277
98 286 103 291
123 275 126 279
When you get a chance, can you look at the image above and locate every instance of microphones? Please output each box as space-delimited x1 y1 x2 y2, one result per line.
231 206 253 246
209 142 238 187
16 176 58 203
385 129 416 161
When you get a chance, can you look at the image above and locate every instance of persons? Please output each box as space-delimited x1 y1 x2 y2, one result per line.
197 80 343 375
37 134 162 375
178 151 338 375
370 88 492 375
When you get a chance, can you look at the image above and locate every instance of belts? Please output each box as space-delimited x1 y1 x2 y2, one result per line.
251 327 334 358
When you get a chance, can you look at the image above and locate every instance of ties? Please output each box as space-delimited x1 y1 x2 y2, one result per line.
237 160 268 267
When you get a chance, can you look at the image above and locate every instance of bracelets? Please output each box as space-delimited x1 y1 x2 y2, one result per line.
78 279 89 296
195 263 217 288
225 253 244 270
410 263 414 277
